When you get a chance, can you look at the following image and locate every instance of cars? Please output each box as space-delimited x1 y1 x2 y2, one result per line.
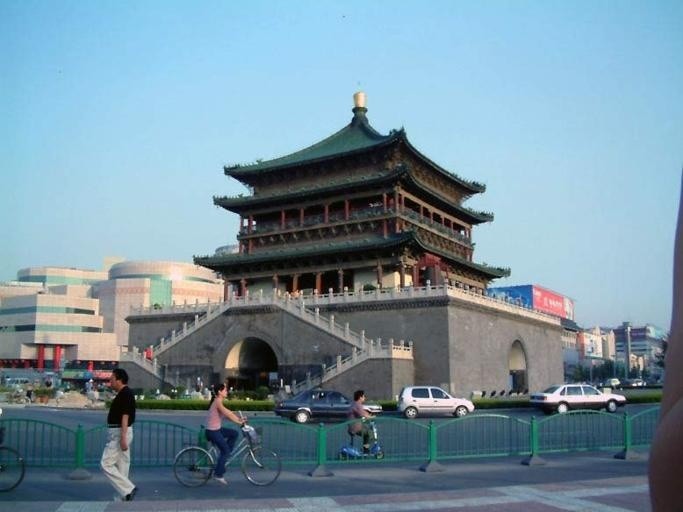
397 386 475 420
273 389 383 425
529 384 627 416
605 378 647 387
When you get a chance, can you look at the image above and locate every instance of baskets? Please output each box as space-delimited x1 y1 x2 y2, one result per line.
242 427 263 445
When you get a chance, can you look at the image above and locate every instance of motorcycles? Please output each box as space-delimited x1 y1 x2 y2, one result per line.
337 412 384 461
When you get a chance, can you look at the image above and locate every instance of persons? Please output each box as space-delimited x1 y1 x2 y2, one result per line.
99 368 138 502
346 390 376 454
205 384 248 486
649 167 683 512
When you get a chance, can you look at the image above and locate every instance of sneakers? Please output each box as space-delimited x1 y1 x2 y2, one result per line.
214 477 228 485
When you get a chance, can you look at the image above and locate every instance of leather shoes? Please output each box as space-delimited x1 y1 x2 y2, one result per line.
126 488 136 501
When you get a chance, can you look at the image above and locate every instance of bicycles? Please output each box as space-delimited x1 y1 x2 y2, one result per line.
0 407 26 493
172 417 282 489
